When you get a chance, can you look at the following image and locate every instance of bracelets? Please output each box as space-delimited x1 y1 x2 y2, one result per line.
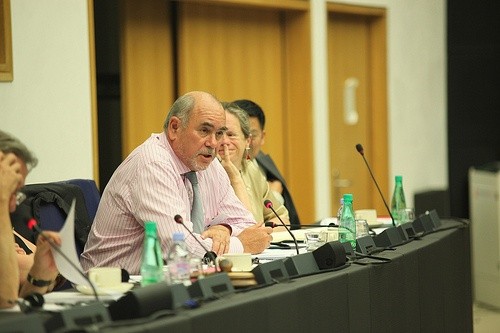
27 273 53 286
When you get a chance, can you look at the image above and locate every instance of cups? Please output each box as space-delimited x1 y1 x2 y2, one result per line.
215 253 252 273
321 228 339 242
305 232 328 252
88 266 121 288
356 209 377 224
405 208 415 223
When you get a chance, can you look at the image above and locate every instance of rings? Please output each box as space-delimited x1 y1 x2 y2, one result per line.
267 243 270 248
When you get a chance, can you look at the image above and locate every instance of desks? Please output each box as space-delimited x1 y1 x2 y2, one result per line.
0 218 475 333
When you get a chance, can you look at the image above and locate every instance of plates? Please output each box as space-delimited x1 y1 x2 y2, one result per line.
368 223 384 228
76 282 134 296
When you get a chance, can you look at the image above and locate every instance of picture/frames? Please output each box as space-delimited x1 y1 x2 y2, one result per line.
0 0 14 82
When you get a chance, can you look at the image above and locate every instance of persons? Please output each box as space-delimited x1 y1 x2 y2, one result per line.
216 100 300 231
0 130 61 312
80 91 273 276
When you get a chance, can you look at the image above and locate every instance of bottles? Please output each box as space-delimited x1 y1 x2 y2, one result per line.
141 221 163 288
338 193 356 251
355 219 370 239
390 176 406 227
167 233 190 285
337 198 345 224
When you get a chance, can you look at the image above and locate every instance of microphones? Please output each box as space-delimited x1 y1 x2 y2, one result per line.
174 215 233 302
24 217 112 333
355 143 417 243
265 222 376 256
264 200 321 278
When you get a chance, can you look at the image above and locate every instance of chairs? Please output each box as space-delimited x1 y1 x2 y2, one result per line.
8 178 101 292
